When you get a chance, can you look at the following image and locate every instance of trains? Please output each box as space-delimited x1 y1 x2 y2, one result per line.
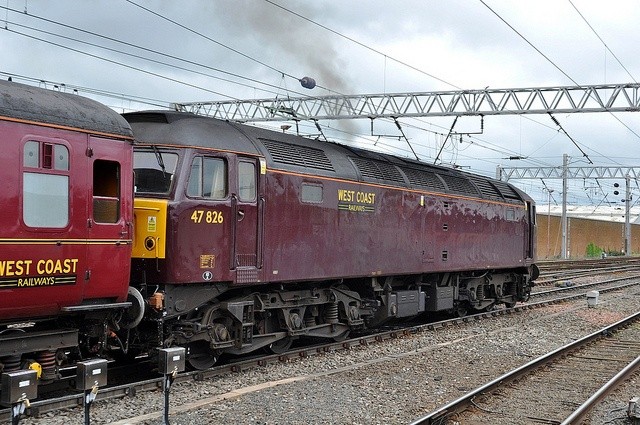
1 79 540 408
